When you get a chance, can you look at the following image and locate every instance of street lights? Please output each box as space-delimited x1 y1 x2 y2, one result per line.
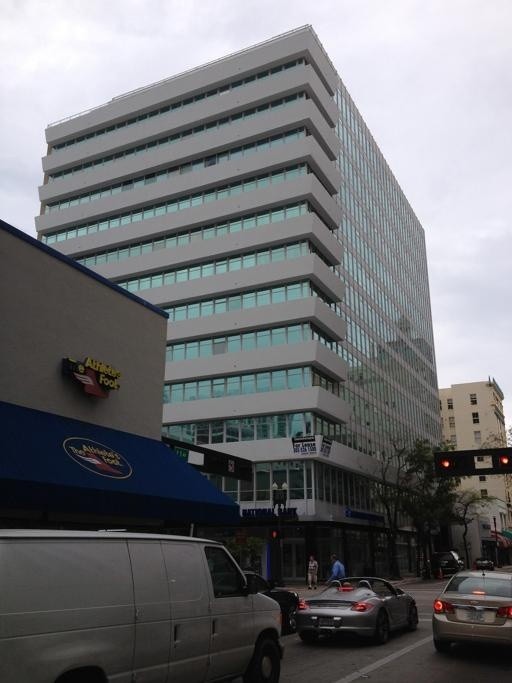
271 480 289 588
492 514 499 567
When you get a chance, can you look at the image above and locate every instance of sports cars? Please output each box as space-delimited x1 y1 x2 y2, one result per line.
292 575 419 647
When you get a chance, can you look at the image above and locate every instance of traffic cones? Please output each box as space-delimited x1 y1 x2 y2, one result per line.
436 566 444 582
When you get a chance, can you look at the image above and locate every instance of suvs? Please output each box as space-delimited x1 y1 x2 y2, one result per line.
431 550 464 575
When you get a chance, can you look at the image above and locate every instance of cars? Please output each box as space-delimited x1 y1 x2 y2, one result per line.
240 569 303 635
474 556 495 571
431 567 512 656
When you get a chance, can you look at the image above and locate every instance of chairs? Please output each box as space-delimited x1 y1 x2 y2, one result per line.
331 579 373 591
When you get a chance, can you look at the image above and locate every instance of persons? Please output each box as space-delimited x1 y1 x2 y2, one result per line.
324 552 346 585
306 553 319 589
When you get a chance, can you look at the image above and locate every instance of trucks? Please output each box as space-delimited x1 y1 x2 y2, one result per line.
0 522 288 683
